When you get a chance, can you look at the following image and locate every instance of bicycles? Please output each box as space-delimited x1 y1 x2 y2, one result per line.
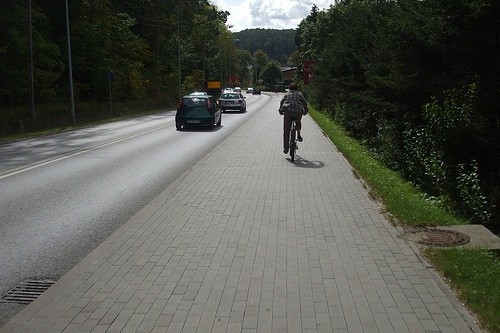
287 119 301 162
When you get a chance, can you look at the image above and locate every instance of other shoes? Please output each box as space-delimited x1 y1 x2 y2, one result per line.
284 145 289 154
298 135 302 142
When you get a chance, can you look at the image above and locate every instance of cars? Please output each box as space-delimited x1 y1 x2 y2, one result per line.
247 88 253 93
218 92 247 113
233 87 242 92
284 86 289 92
175 92 223 130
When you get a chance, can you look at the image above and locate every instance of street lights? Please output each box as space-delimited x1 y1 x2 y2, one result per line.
220 39 239 90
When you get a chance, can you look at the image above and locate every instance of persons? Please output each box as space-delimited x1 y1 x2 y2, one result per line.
279 83 308 155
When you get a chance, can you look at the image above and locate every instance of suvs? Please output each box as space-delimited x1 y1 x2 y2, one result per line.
252 86 261 95
